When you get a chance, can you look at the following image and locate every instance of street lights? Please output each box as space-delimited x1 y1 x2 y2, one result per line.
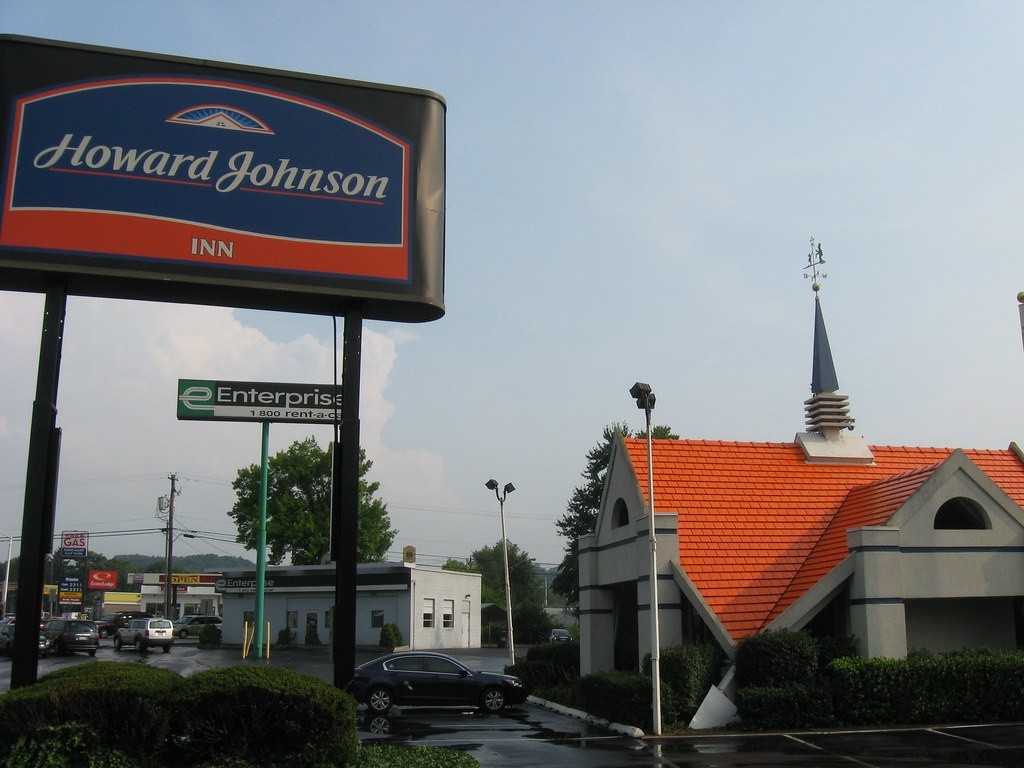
629 382 662 732
486 478 516 665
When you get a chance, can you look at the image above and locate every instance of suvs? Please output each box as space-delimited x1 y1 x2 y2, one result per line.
112 618 174 655
40 617 100 657
93 611 156 639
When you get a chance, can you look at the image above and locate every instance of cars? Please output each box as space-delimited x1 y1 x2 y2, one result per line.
172 614 222 639
350 651 530 717
549 629 573 642
0 625 50 658
0 617 47 631
